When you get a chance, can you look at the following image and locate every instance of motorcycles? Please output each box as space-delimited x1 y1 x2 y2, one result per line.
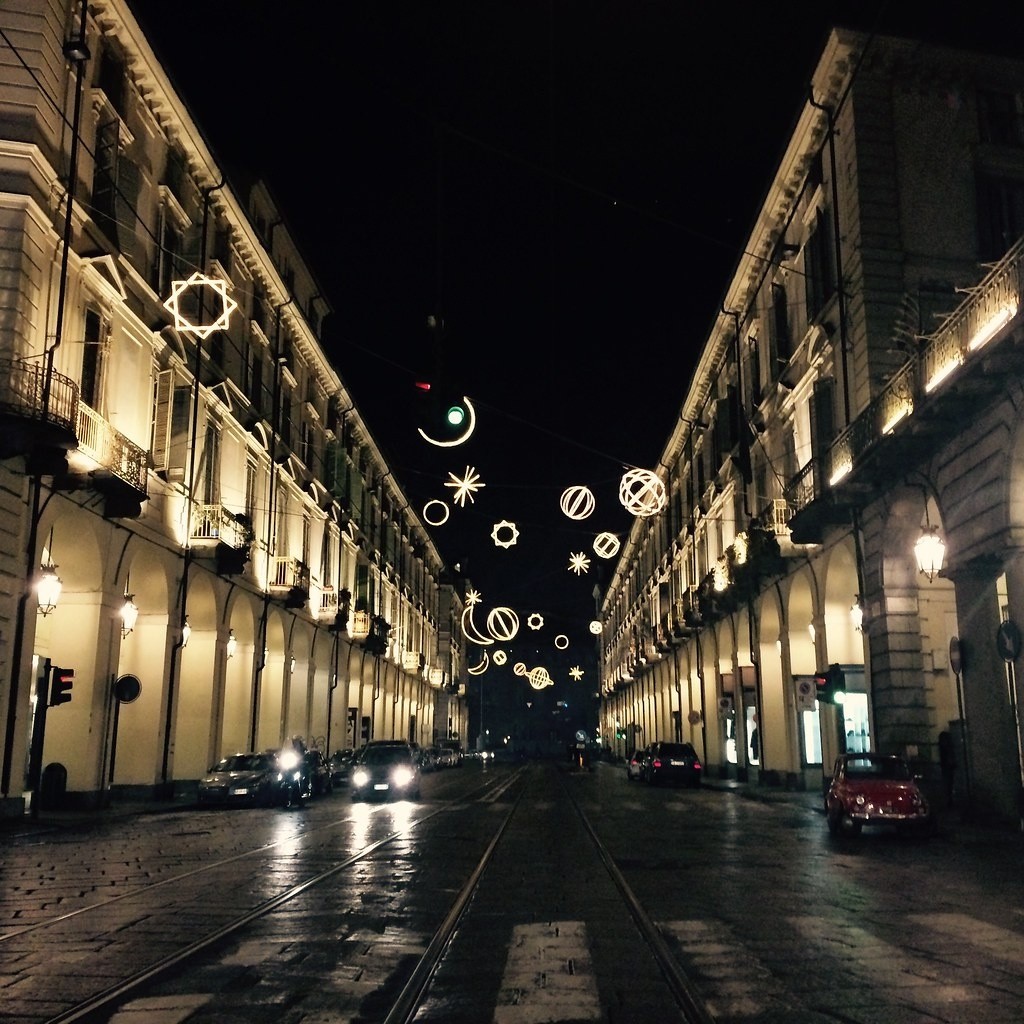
275 736 311 810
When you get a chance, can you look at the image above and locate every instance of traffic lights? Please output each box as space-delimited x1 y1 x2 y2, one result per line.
50 668 74 706
617 729 620 739
833 670 845 704
814 671 832 704
445 390 466 427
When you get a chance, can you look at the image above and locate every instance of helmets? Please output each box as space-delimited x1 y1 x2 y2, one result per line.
292 734 305 748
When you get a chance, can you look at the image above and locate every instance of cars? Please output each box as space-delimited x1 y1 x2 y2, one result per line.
198 753 304 809
329 748 353 788
628 749 645 778
419 746 462 772
826 753 932 843
303 749 332 800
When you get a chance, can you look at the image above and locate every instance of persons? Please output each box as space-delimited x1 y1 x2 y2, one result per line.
280 735 310 794
938 718 964 808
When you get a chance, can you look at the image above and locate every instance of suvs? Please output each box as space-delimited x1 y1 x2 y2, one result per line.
642 742 702 784
351 738 421 802
